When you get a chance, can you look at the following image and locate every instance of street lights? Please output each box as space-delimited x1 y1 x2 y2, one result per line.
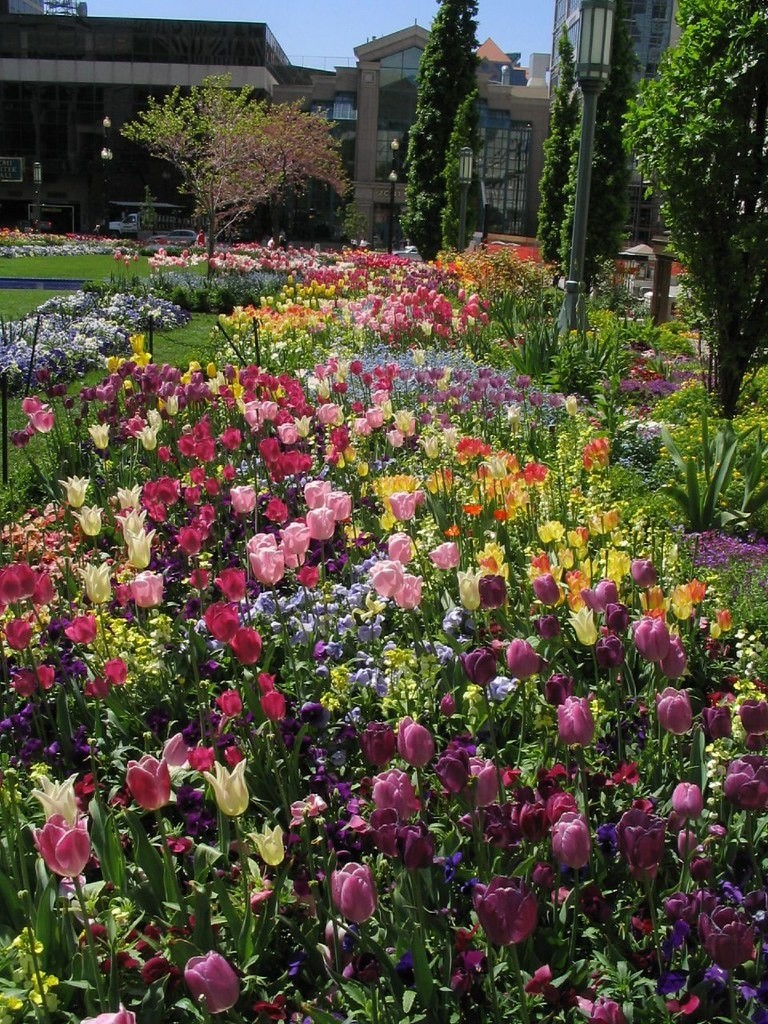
388 139 399 255
100 117 114 236
558 0 620 331
459 147 475 250
33 162 42 233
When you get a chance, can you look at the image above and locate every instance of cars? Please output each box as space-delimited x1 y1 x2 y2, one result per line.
149 230 197 247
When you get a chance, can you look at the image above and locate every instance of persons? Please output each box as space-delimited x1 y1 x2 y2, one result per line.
92 224 101 235
267 237 275 250
196 229 207 246
314 240 321 252
281 236 287 250
279 231 285 244
360 237 369 247
352 238 357 250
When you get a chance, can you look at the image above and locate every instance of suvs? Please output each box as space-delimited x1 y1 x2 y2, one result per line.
391 246 422 260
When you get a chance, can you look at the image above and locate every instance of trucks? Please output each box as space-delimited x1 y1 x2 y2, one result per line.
110 213 170 240
